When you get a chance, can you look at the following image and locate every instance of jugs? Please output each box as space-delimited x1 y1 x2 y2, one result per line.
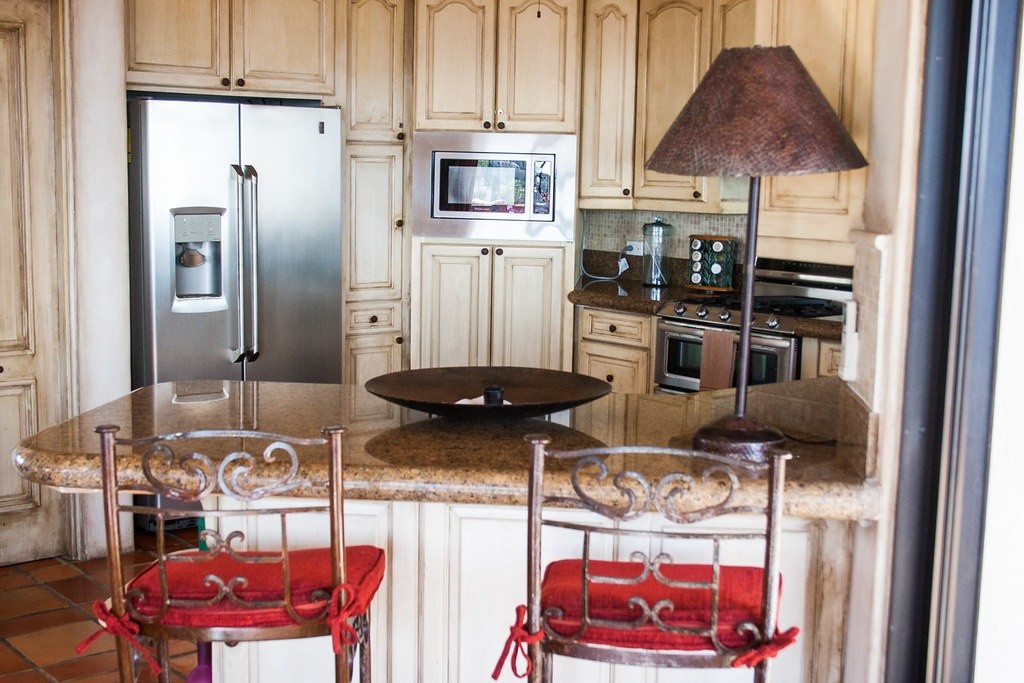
643 217 671 287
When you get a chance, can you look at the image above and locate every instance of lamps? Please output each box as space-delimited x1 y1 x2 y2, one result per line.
643 45 870 464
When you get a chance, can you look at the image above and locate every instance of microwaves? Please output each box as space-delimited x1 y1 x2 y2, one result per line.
432 152 554 221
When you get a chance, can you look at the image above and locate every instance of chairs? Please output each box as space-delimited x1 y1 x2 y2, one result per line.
94 422 387 683
525 435 796 683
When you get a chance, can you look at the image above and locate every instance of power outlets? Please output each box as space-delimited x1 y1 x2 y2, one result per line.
626 240 645 256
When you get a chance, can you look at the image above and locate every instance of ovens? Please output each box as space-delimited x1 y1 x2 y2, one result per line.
655 319 797 394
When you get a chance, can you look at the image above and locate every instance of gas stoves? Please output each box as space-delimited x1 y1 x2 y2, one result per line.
663 292 846 341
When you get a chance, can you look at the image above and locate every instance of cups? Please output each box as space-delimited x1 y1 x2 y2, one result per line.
483 386 503 405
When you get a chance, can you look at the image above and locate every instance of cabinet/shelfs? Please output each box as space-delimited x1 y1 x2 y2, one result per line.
410 235 577 427
580 306 843 394
578 0 638 211
339 0 407 438
412 0 585 131
633 0 877 215
127 0 336 94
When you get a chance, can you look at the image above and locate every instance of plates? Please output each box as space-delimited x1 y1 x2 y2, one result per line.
365 367 611 419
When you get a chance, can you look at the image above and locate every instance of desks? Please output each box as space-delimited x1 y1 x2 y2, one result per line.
14 379 886 683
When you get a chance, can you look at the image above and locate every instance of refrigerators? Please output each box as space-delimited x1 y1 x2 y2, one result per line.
128 98 343 531
